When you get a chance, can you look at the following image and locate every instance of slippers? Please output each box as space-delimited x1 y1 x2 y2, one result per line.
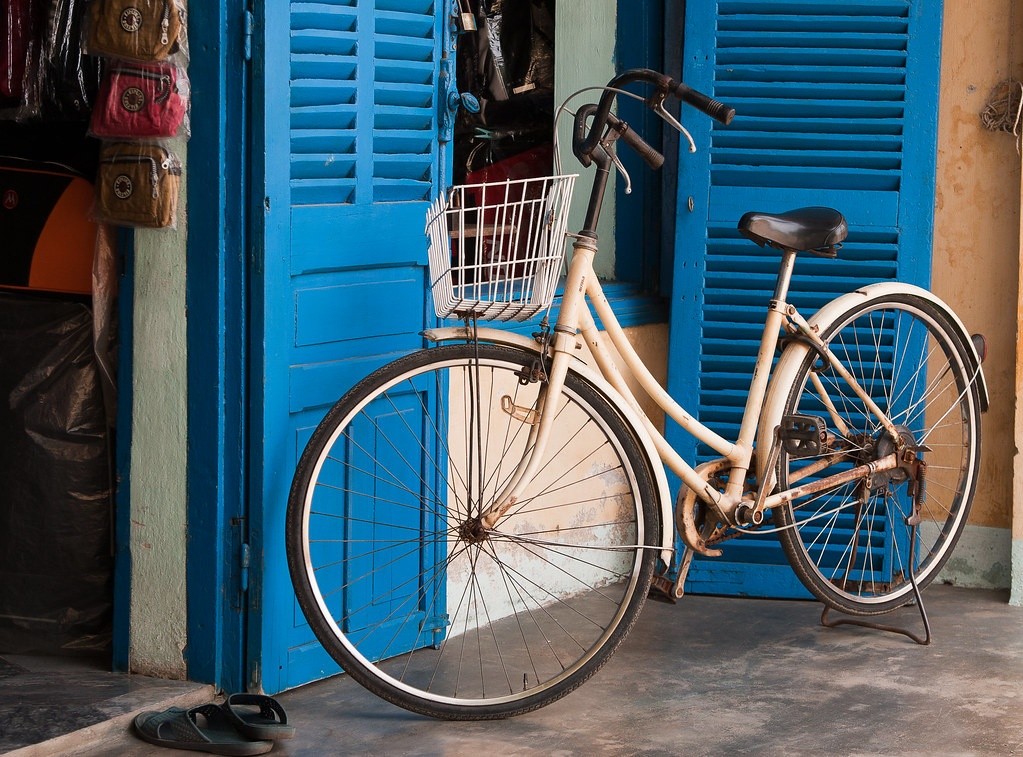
134 693 299 755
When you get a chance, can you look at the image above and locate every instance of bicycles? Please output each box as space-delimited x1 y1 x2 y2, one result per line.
283 63 988 724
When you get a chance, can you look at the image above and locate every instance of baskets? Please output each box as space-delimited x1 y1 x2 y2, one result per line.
426 170 577 320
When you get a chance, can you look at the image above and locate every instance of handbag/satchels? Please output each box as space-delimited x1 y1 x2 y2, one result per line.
94 0 184 227
